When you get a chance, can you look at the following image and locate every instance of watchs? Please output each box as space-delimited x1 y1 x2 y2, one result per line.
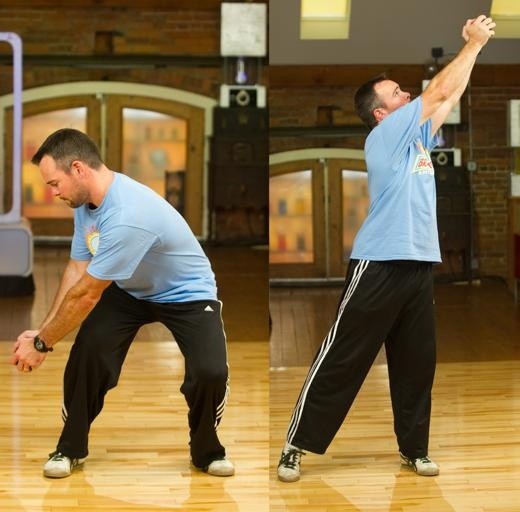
33 335 53 353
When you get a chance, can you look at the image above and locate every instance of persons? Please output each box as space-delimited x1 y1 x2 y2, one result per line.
12 126 239 479
276 13 496 485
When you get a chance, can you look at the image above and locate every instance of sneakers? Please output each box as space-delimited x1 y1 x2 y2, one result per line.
42 450 89 478
400 450 440 476
277 441 303 483
189 455 236 477
